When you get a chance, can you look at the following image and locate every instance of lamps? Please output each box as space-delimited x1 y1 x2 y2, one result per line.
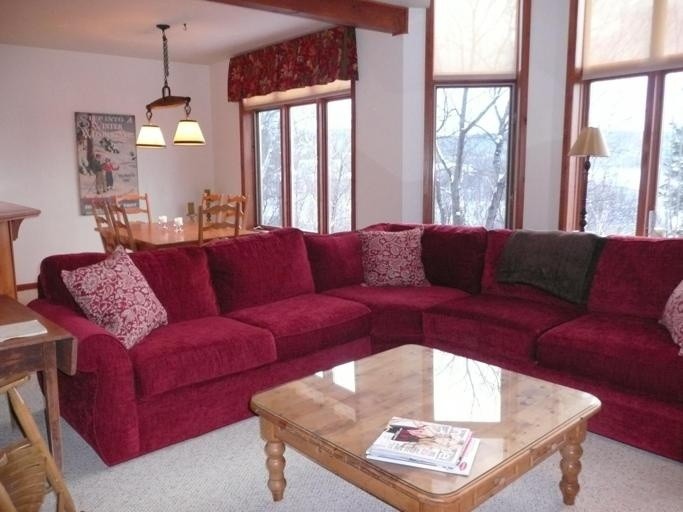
567 127 611 233
135 24 206 148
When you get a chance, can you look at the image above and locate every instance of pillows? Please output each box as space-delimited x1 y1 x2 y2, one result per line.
61 244 169 352
359 228 431 289
660 279 683 353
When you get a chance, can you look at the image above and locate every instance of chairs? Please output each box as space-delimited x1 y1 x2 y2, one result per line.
91 190 248 251
0 377 76 512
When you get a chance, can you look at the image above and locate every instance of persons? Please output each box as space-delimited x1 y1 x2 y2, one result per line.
90 153 107 194
102 154 120 192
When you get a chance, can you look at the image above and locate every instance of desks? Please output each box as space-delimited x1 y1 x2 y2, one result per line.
0 292 78 471
0 201 51 298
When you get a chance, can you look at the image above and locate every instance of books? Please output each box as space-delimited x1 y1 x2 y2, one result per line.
365 414 480 477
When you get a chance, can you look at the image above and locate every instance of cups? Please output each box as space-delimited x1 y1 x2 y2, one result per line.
157 215 168 229
172 217 183 232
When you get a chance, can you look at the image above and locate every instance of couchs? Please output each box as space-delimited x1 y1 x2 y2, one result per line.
423 223 683 462
28 223 487 469
